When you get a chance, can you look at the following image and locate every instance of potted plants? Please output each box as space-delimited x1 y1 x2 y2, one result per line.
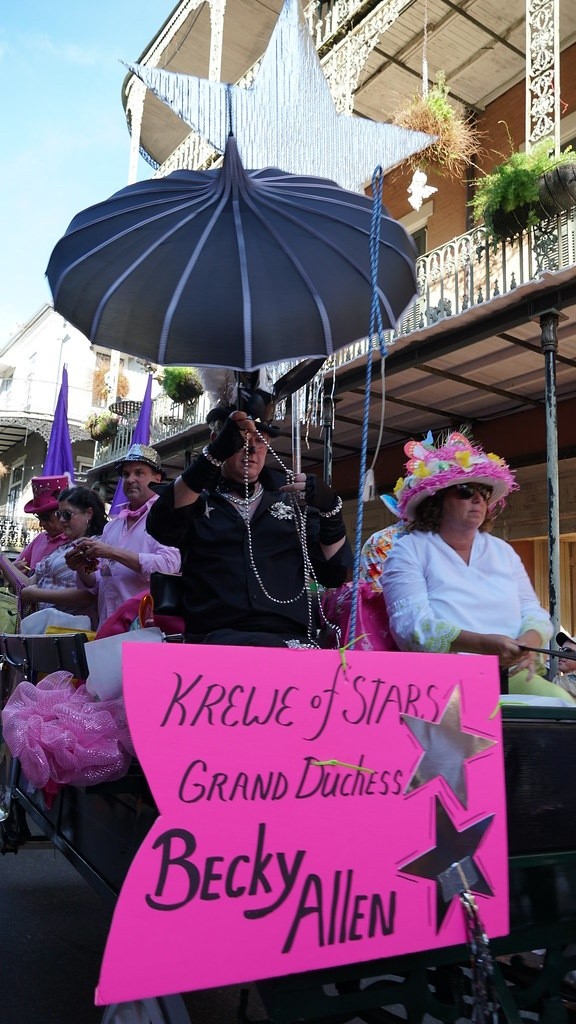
458 118 576 250
155 366 202 404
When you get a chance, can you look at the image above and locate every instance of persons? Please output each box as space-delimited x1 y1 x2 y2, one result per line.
360 518 408 591
0 486 107 634
380 432 573 701
147 388 353 648
65 445 180 633
554 632 576 699
1 476 68 590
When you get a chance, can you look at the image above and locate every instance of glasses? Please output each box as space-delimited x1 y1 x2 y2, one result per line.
35 508 59 522
55 510 91 521
558 647 576 655
452 483 492 502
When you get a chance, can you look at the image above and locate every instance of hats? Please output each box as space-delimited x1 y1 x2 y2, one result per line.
201 359 324 438
393 431 518 524
556 631 576 646
114 444 167 481
23 475 70 513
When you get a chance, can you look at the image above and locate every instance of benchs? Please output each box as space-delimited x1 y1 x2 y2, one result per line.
1 587 397 710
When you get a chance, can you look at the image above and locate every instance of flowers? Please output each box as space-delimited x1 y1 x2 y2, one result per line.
78 409 118 432
394 426 522 523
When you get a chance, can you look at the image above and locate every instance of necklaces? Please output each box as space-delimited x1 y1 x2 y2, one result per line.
244 429 342 648
0 558 38 634
215 484 264 505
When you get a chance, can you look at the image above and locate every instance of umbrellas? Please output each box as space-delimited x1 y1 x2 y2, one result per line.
46 132 419 368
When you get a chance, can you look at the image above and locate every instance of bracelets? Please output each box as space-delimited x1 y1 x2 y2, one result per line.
202 446 225 467
319 496 343 517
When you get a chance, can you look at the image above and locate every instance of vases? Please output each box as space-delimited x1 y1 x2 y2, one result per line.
89 420 116 441
